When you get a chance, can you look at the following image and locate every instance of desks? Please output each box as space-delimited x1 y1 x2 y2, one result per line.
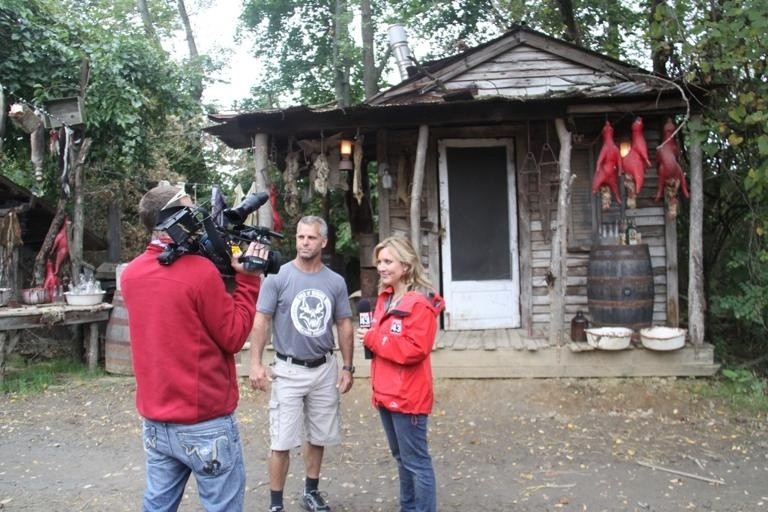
0 294 114 378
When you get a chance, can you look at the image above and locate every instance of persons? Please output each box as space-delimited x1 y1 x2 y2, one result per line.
119 185 272 512
249 214 353 512
357 236 447 512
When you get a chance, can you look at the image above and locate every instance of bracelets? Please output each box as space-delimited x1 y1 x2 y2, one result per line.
343 366 355 375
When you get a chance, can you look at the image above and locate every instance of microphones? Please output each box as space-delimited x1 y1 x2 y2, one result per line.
355 300 377 361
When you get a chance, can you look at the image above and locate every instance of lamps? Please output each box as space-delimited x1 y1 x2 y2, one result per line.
620 119 632 175
339 128 354 171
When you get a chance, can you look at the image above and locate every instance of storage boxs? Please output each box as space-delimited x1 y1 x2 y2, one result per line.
42 96 88 129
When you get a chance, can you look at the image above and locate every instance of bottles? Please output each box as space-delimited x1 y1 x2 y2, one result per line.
599 217 641 245
570 311 589 342
52 273 107 307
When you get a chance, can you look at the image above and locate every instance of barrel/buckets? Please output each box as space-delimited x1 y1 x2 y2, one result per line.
587 244 654 339
104 290 135 375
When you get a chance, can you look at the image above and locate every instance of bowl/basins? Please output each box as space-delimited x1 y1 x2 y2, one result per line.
584 326 632 352
0 287 12 307
22 288 46 306
640 327 687 351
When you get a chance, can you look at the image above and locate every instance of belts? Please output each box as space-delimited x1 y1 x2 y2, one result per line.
276 349 333 367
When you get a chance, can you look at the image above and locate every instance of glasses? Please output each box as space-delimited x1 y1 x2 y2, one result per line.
161 185 187 211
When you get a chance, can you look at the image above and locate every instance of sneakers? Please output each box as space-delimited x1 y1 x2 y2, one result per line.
269 505 284 512
300 488 331 512
